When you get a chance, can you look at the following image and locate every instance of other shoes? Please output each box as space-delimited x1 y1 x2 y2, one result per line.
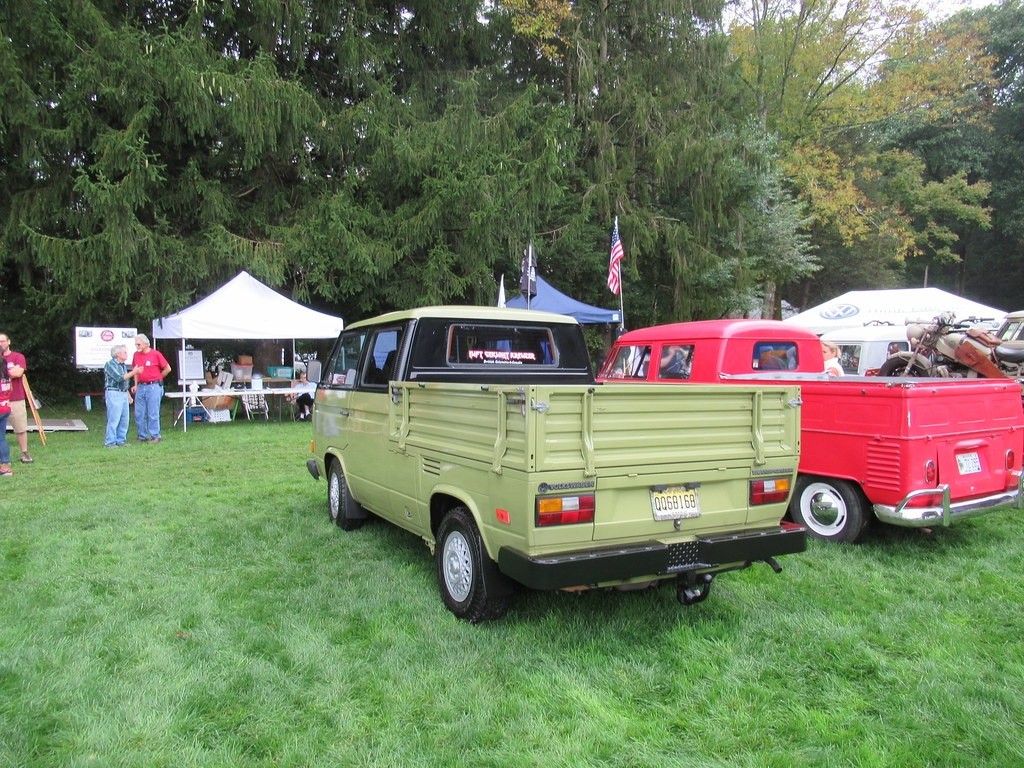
148 438 161 444
105 445 119 449
306 414 312 420
298 418 305 422
133 438 144 443
120 442 131 447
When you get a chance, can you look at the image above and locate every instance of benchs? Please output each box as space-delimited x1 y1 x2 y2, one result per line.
77 392 106 411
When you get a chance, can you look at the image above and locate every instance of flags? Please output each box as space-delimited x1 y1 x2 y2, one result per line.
606 222 624 294
519 238 537 299
498 274 507 308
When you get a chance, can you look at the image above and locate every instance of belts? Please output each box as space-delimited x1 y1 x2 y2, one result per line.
138 380 159 385
104 388 127 392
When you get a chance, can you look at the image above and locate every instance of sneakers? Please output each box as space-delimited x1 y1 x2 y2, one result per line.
0 468 14 477
20 452 34 463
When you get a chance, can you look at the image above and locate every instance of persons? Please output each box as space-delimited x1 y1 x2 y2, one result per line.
103 344 144 447
286 372 317 423
130 334 171 443
757 340 845 377
660 346 693 380
0 356 13 476
0 334 34 462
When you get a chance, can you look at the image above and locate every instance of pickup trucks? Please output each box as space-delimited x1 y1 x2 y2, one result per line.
306 305 805 622
594 320 1024 542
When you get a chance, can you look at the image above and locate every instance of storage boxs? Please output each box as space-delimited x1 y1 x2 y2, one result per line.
266 367 292 379
186 355 254 422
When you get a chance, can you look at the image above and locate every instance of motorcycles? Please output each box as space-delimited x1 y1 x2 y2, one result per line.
877 310 1024 380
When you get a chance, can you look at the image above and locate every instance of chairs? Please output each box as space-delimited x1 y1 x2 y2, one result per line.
242 390 269 421
290 381 314 422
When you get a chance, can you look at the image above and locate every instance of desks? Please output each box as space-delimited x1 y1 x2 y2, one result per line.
164 378 316 426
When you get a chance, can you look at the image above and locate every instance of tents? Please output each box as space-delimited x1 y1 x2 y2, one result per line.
491 275 622 366
152 270 344 432
778 286 1023 377
